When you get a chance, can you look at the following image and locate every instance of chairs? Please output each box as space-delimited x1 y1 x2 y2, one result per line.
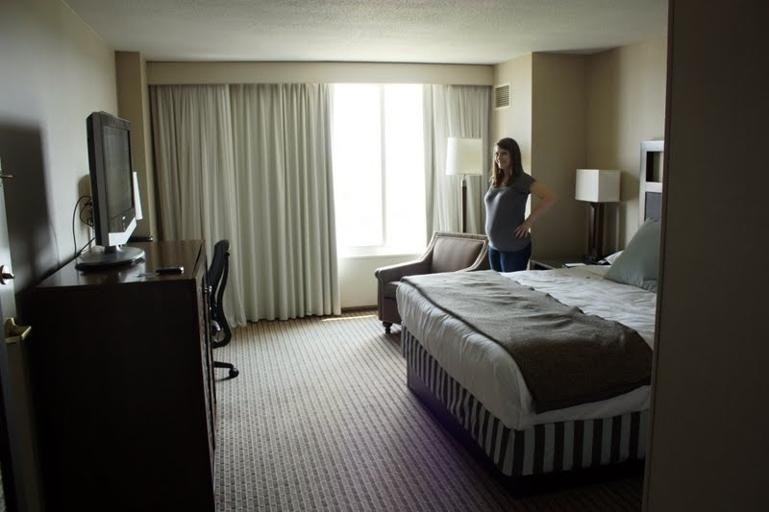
374 231 489 334
208 240 239 378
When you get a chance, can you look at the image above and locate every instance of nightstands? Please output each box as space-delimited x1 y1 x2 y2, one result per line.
532 255 595 271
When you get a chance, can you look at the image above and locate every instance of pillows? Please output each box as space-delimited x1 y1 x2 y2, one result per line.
605 216 661 293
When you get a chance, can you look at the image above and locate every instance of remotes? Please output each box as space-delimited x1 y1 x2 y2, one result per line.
155 264 184 273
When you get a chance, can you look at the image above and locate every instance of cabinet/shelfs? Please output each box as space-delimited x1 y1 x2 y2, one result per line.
23 240 216 512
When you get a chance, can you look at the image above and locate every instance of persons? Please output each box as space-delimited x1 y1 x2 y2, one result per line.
483 137 558 271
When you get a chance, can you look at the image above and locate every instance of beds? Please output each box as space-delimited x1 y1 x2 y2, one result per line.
395 141 665 499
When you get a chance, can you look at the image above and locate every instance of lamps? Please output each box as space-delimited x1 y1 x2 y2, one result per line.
574 168 621 264
445 137 482 234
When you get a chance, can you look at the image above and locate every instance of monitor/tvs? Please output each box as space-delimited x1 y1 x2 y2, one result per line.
76 111 146 270
